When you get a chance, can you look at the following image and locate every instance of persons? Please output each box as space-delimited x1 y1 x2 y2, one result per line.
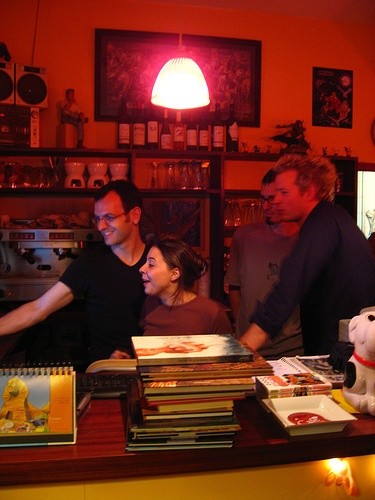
241 154 375 357
228 170 305 357
61 88 89 148
110 234 233 360
0 179 151 360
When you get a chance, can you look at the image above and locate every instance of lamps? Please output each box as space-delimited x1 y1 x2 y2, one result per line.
150 0 210 110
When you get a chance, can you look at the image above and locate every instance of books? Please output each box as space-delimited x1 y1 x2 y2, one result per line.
123 334 275 452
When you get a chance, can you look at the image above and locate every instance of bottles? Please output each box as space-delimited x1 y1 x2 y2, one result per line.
132 100 145 149
226 103 240 152
160 109 172 151
199 112 209 152
185 111 198 151
116 99 131 149
172 111 186 151
147 107 160 149
211 103 226 152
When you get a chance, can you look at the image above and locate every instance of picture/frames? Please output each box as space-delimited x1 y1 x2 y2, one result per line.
93 27 262 127
312 66 353 128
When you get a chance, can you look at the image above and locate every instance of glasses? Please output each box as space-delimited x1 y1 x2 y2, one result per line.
91 212 125 225
256 192 275 204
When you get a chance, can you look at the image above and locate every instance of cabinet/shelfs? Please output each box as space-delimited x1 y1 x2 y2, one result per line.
0 147 358 338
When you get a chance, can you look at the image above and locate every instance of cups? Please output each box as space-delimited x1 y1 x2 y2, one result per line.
63 161 128 189
0 162 55 190
151 161 211 190
224 198 268 226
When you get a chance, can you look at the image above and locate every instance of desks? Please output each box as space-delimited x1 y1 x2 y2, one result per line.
0 382 375 500
